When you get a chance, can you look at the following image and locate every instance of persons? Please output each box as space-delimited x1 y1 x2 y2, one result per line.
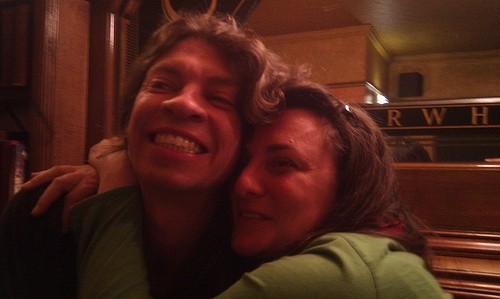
386 134 432 164
0 5 316 299
21 77 457 299
